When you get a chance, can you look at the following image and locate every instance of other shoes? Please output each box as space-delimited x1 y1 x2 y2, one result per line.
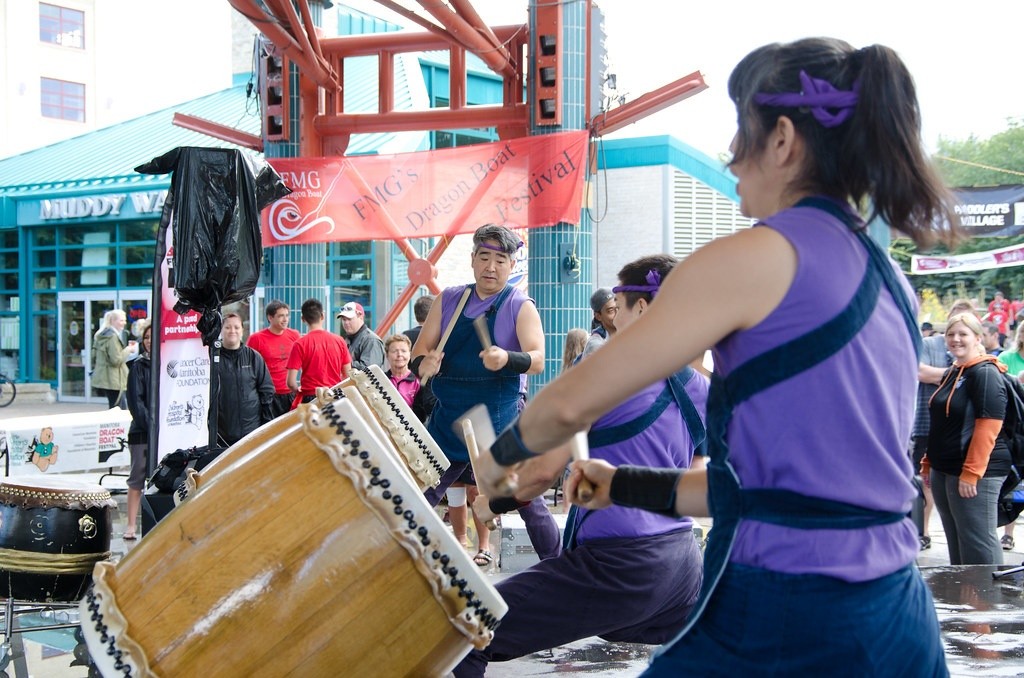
442 508 451 526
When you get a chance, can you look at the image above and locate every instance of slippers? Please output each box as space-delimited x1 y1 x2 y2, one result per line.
122 533 138 540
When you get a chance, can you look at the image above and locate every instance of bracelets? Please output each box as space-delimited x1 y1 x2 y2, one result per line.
411 355 425 374
489 415 542 467
489 490 531 515
609 464 689 519
502 351 532 374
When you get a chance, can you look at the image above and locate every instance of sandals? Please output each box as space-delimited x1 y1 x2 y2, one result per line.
472 549 494 565
999 535 1015 549
919 536 932 549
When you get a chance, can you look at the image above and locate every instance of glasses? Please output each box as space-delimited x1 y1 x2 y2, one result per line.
339 306 353 312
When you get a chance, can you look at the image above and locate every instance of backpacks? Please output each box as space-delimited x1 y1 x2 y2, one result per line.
970 361 1024 477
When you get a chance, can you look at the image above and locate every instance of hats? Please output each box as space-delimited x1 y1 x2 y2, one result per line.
993 291 1004 296
589 287 615 312
921 322 934 330
336 301 364 319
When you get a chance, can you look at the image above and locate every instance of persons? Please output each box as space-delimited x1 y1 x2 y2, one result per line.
400 224 563 561
217 298 494 566
93 309 139 409
910 291 1024 566
463 38 959 678
123 324 152 540
562 286 617 514
450 255 711 678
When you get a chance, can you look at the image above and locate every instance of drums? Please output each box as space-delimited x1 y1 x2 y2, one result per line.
76 395 511 676
329 362 452 498
0 471 120 603
170 383 422 507
